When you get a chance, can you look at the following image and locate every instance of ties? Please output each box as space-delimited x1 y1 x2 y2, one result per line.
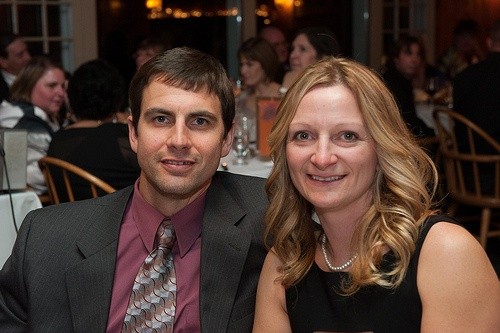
118 220 177 333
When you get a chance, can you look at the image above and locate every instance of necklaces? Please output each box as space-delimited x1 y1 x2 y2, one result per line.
322 228 359 269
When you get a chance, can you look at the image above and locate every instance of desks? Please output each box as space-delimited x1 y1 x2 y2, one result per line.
216 131 321 224
0 188 43 269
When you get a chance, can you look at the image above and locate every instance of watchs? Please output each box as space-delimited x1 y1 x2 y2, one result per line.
278 86 287 98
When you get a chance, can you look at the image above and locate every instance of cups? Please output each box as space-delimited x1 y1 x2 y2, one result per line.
231 113 253 165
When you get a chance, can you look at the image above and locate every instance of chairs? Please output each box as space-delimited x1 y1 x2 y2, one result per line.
432 106 500 248
37 157 116 204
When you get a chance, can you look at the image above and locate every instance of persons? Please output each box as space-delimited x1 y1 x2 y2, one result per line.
237 23 337 137
252 57 500 333
0 31 173 207
0 46 271 333
380 17 500 254
